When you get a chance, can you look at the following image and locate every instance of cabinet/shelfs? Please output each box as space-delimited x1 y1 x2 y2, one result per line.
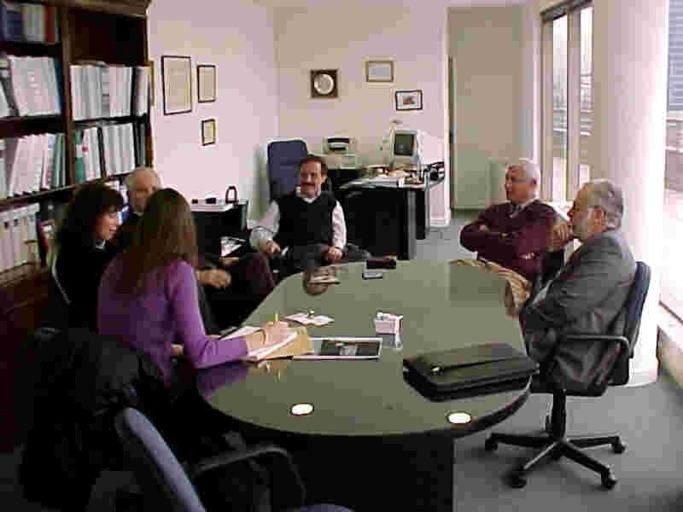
1 275 55 454
0 0 154 269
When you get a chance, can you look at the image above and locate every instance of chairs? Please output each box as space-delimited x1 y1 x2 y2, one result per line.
479 258 653 495
113 405 359 511
265 137 333 207
31 324 150 419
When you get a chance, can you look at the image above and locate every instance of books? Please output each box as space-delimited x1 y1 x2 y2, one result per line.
0 0 67 273
68 64 149 182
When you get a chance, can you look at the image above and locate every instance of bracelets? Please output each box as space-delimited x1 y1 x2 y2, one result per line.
195 270 202 283
255 328 266 344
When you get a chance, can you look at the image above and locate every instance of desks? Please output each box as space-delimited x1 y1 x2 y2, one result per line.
194 253 538 512
190 198 250 254
326 164 447 263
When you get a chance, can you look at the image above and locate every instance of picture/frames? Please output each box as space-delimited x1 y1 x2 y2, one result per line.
158 52 194 117
196 62 217 103
200 118 217 147
308 68 339 101
393 88 423 112
363 59 396 84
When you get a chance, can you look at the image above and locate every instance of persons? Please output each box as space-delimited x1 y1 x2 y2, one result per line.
247 157 371 269
457 158 575 305
103 168 237 288
46 181 118 345
519 183 638 394
95 189 292 402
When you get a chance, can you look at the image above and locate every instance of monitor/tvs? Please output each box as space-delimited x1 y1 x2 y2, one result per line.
390 128 423 170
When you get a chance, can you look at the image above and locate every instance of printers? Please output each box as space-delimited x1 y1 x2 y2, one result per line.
321 135 357 154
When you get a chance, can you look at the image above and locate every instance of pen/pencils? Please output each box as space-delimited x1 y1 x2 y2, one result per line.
273 310 278 324
